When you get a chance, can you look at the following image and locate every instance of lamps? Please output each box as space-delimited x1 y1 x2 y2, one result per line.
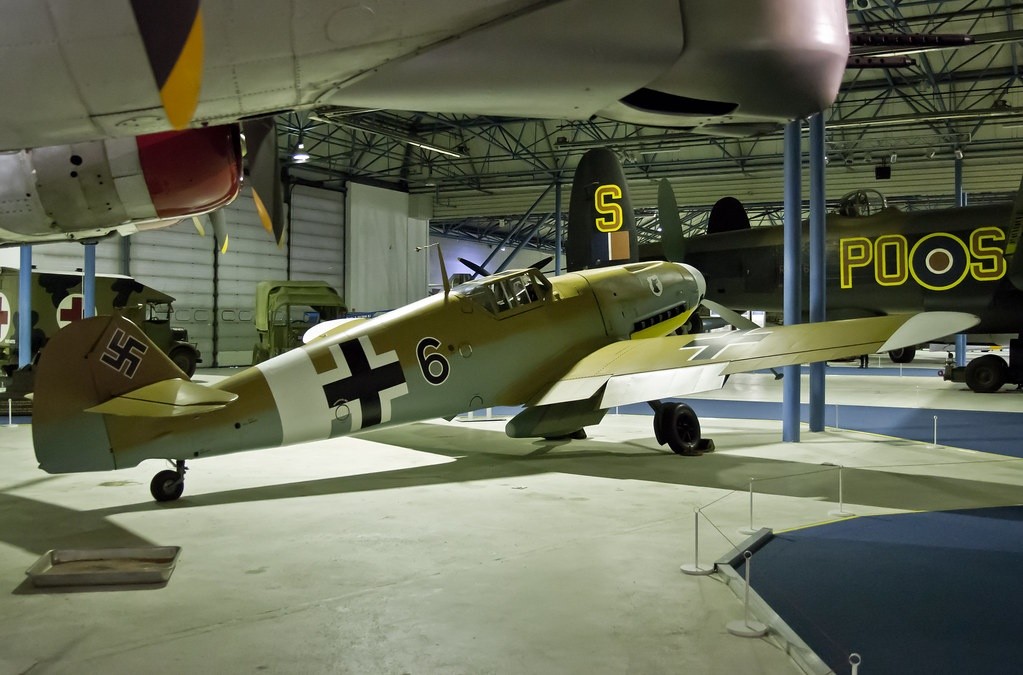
864 153 872 163
846 155 854 164
954 150 964 160
890 153 899 164
292 144 310 161
927 149 936 159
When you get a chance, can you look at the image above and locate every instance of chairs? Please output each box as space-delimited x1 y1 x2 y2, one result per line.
472 287 515 312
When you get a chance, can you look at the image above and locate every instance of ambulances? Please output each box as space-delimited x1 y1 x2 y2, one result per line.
0 267 204 382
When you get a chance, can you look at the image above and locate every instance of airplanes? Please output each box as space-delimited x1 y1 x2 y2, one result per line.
564 149 1023 396
30 242 982 504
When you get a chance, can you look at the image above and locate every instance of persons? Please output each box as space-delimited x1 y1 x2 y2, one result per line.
858 354 869 368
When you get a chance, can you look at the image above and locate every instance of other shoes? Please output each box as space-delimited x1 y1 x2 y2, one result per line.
865 366 868 368
859 366 863 368
